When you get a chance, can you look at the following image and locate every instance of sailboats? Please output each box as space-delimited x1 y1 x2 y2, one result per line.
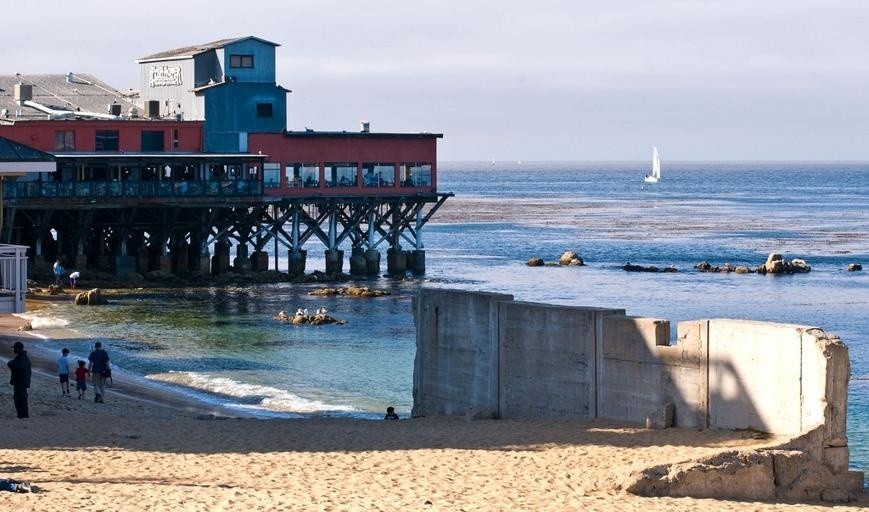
644 145 661 183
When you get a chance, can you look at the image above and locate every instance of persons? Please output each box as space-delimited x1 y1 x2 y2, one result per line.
7 341 32 418
74 360 90 400
303 165 386 187
53 259 83 290
89 341 111 403
384 406 400 420
56 348 72 398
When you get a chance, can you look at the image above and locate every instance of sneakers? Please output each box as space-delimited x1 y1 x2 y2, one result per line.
61 390 104 403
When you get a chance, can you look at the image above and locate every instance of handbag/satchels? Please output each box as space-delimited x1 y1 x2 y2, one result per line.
102 363 111 379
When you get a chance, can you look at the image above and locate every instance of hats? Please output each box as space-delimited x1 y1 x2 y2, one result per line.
10 341 24 349
